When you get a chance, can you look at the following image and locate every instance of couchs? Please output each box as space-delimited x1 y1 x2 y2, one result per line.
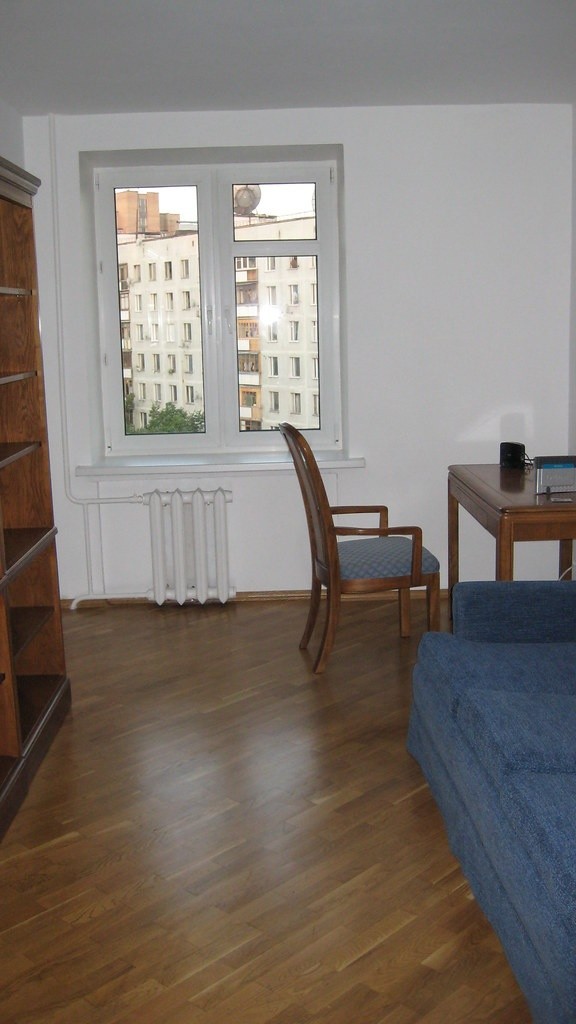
404 581 576 1024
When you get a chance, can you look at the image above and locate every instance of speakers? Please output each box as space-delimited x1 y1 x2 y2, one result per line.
500 442 525 468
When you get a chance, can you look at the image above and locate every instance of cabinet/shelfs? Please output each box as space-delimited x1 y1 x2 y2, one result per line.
0 154 74 839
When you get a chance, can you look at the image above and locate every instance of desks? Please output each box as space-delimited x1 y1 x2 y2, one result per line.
447 463 576 634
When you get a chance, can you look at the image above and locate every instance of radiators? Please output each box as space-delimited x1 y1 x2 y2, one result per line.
141 487 236 606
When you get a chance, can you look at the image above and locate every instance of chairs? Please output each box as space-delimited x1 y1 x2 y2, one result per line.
278 422 441 675
